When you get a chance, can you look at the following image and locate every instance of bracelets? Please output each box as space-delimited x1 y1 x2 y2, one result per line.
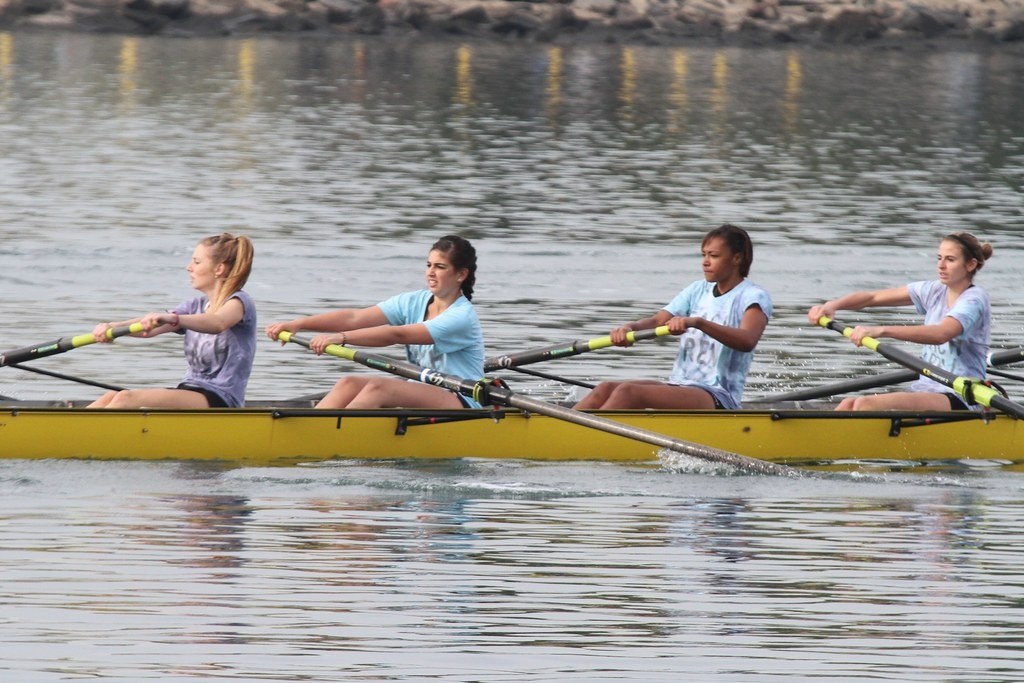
341 332 346 347
170 311 179 327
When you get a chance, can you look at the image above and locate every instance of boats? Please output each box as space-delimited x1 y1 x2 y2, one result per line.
0 397 1024 465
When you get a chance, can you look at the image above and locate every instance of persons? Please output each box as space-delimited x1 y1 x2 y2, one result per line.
85 234 257 409
808 232 992 410
267 235 485 409
572 224 771 410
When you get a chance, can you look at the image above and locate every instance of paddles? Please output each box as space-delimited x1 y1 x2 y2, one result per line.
278 331 795 475
484 324 685 372
0 316 167 367
818 316 1023 421
755 347 1023 403
